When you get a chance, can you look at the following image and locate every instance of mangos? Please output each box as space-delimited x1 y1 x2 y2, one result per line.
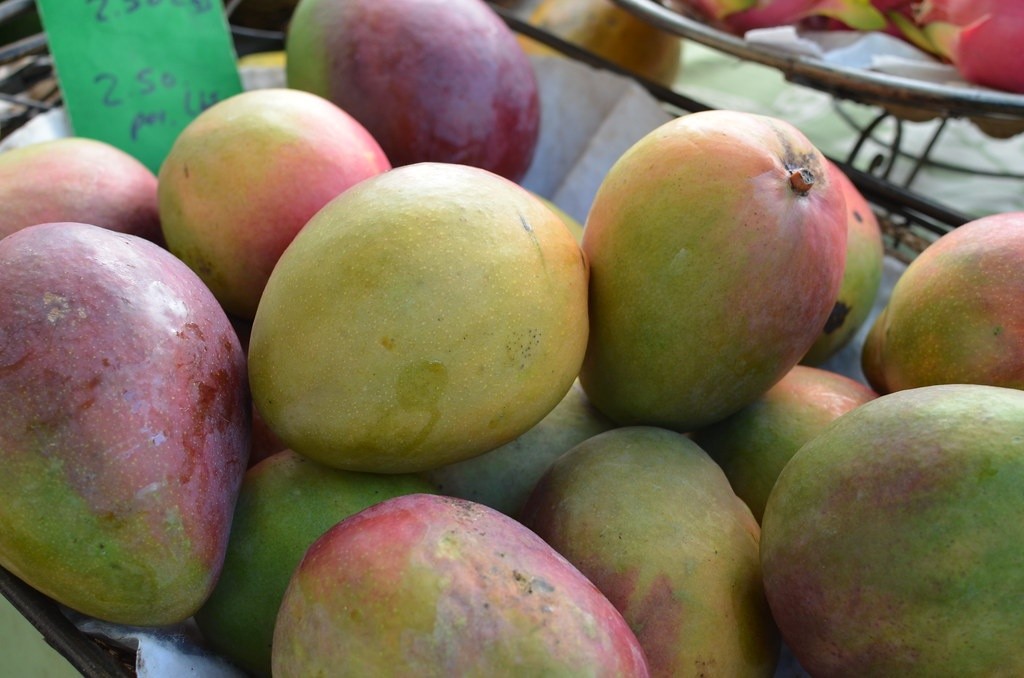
0 0 1024 678
